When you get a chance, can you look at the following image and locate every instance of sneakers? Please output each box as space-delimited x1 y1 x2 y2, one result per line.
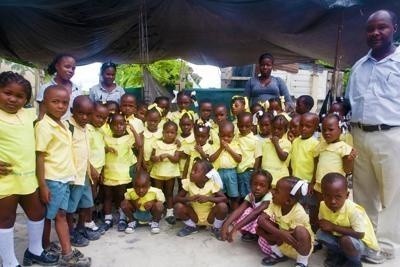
241 233 257 241
364 250 394 264
179 225 198 236
211 227 223 240
23 219 159 267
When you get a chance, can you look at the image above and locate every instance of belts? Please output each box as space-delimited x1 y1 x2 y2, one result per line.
357 122 400 132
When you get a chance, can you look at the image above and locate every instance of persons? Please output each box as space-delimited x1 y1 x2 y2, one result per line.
0 71 92 266
257 176 315 267
345 8 400 266
89 60 128 106
65 95 356 246
244 53 293 107
315 173 380 266
35 53 84 117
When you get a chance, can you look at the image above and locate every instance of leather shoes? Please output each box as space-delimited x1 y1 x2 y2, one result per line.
263 253 288 265
